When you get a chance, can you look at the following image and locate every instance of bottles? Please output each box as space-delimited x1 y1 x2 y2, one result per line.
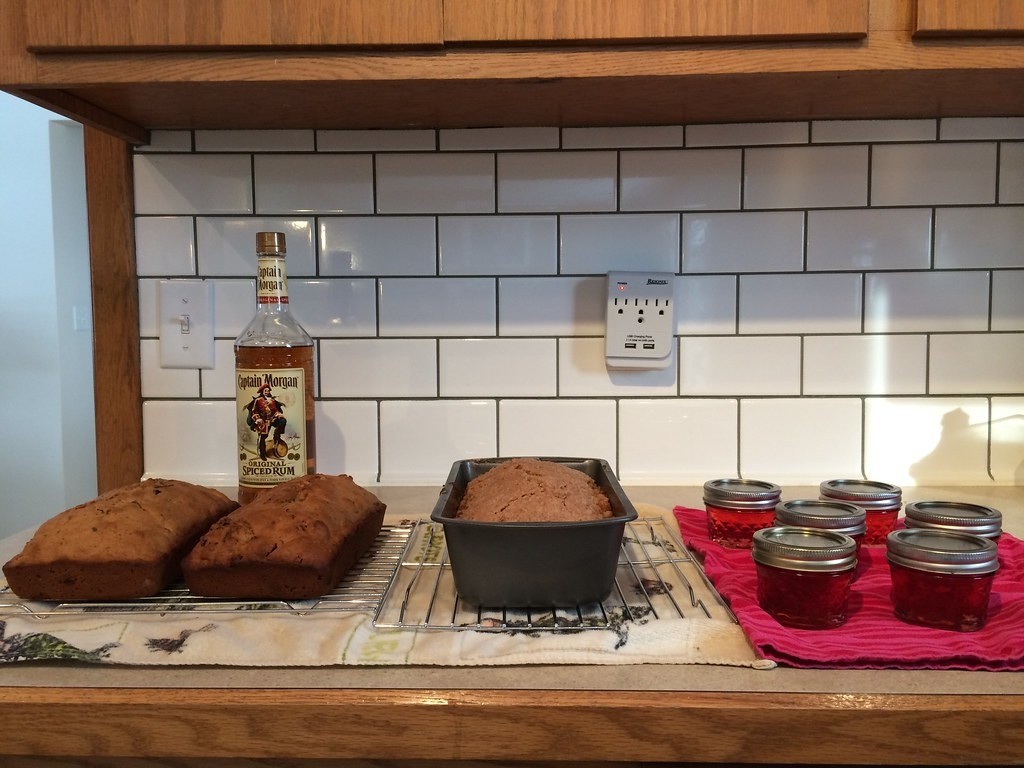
235 233 318 503
708 480 1001 632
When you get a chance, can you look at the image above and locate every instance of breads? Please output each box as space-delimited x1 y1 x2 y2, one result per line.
454 456 614 521
2 478 241 601
180 474 387 601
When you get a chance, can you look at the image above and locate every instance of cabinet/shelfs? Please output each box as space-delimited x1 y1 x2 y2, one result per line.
0 0 1024 149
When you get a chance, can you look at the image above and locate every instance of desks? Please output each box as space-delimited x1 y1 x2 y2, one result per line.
0 482 1024 768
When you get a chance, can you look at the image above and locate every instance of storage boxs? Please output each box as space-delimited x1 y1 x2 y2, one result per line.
429 457 639 612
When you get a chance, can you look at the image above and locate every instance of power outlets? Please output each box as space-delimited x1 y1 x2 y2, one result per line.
604 270 673 368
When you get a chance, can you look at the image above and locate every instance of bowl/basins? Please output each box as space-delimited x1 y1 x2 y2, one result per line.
430 458 638 610
